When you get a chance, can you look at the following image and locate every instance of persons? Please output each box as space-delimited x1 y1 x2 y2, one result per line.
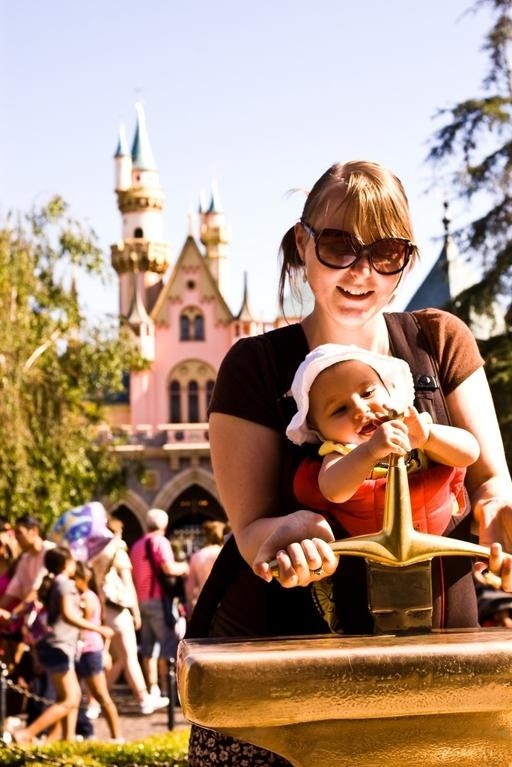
181 161 511 766
283 341 485 629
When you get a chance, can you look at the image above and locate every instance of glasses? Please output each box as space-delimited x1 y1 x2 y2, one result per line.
297 219 413 276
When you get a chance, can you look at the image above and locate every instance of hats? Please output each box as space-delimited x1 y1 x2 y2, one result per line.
285 343 416 447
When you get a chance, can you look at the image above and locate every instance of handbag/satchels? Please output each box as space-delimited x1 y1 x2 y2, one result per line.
162 583 188 630
102 544 138 609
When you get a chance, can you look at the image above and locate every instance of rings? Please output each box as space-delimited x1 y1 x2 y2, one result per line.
310 565 325 576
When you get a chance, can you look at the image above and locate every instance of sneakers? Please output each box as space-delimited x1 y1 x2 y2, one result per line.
0 682 179 747
